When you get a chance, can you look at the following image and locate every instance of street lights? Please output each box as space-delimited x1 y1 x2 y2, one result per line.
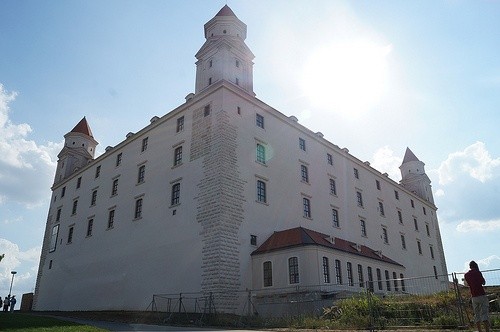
9 272 17 295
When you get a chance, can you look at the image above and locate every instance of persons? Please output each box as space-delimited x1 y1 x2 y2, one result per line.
10 296 16 311
0 296 3 310
464 260 491 332
2 297 10 312
